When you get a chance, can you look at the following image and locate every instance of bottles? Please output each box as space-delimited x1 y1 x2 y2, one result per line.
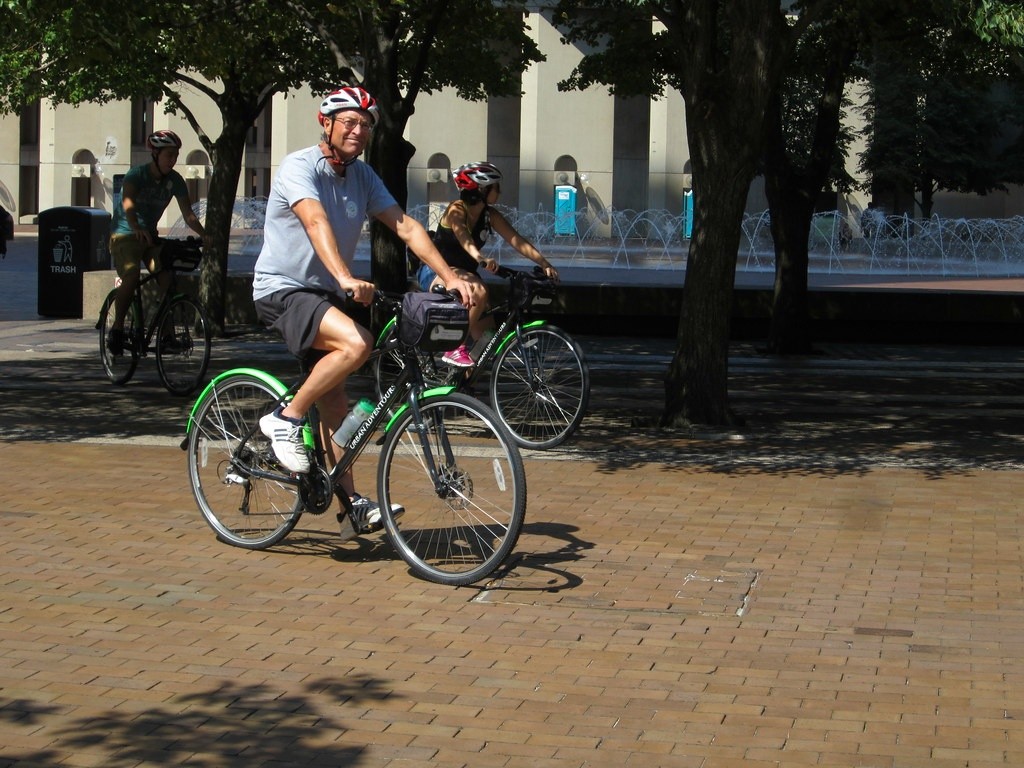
332 396 377 448
468 327 495 362
144 298 160 328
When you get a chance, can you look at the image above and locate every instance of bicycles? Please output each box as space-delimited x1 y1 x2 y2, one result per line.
94 235 212 397
180 284 527 586
372 261 590 451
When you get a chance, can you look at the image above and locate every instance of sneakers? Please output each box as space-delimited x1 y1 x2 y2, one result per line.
337 493 405 541
441 346 475 368
259 406 310 473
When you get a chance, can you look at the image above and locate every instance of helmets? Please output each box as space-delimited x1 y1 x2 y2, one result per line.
452 161 504 193
318 88 381 126
148 130 183 151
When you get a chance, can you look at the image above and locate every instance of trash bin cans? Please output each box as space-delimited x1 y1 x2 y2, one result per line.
37 206 112 319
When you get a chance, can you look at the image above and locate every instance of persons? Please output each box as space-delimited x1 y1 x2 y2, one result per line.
414 161 560 420
860 202 874 238
840 224 853 252
252 88 475 541
106 131 216 357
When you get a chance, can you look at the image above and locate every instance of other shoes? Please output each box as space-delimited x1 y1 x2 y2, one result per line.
108 327 125 358
162 337 184 353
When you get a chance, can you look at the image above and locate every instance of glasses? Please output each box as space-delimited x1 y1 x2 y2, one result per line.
330 117 376 135
